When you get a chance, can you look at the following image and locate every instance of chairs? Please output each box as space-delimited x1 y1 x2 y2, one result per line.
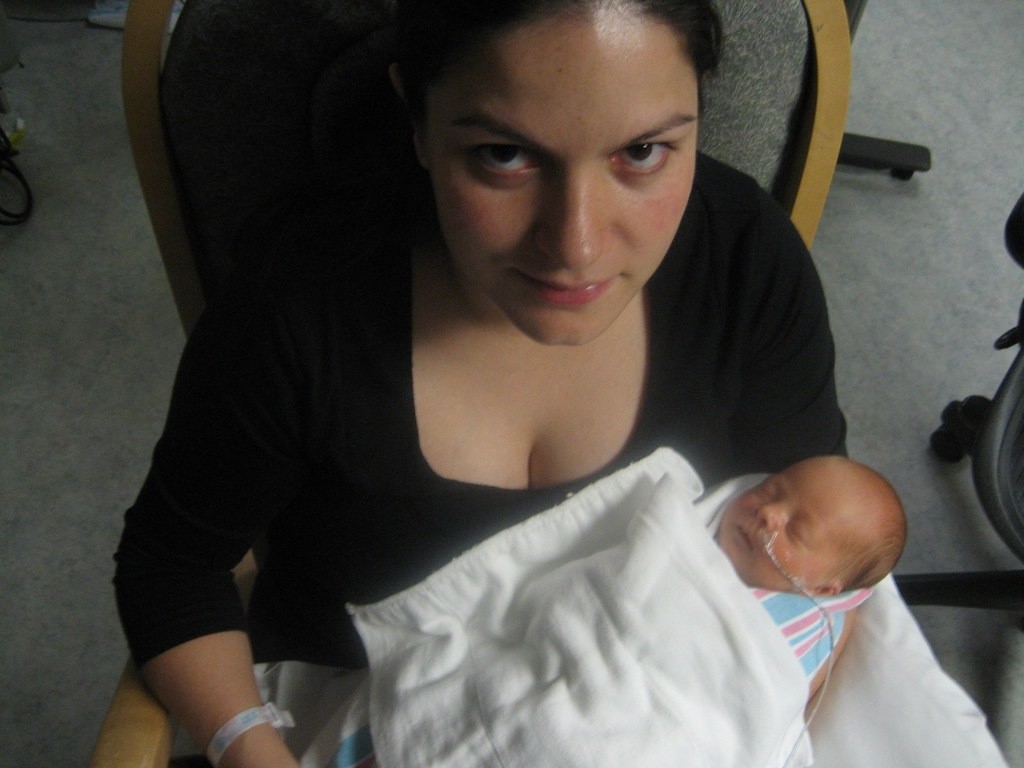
89 0 1009 768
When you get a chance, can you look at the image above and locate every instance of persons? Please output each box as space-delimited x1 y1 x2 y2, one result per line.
111 0 849 768
297 444 907 768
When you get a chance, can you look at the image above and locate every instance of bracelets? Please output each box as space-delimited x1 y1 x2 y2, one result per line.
205 702 296 767
